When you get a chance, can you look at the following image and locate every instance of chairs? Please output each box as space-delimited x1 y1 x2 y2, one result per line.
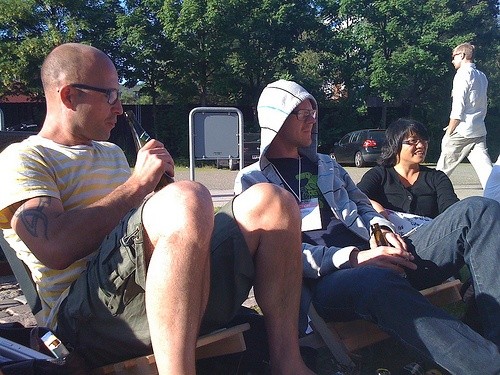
0 230 462 375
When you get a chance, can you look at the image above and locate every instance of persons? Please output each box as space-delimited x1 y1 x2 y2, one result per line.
235 78 500 375
0 44 319 375
357 42 500 302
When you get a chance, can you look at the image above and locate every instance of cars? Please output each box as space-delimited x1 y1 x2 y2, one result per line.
216 132 262 170
326 128 395 167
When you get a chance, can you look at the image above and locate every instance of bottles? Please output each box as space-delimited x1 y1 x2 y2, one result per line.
370 223 393 247
124 110 175 193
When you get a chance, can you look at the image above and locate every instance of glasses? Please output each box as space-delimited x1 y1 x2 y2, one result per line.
58 84 121 104
292 109 317 121
402 137 430 144
452 53 465 60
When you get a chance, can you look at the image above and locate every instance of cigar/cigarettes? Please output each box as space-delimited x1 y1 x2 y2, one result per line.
408 252 412 260
